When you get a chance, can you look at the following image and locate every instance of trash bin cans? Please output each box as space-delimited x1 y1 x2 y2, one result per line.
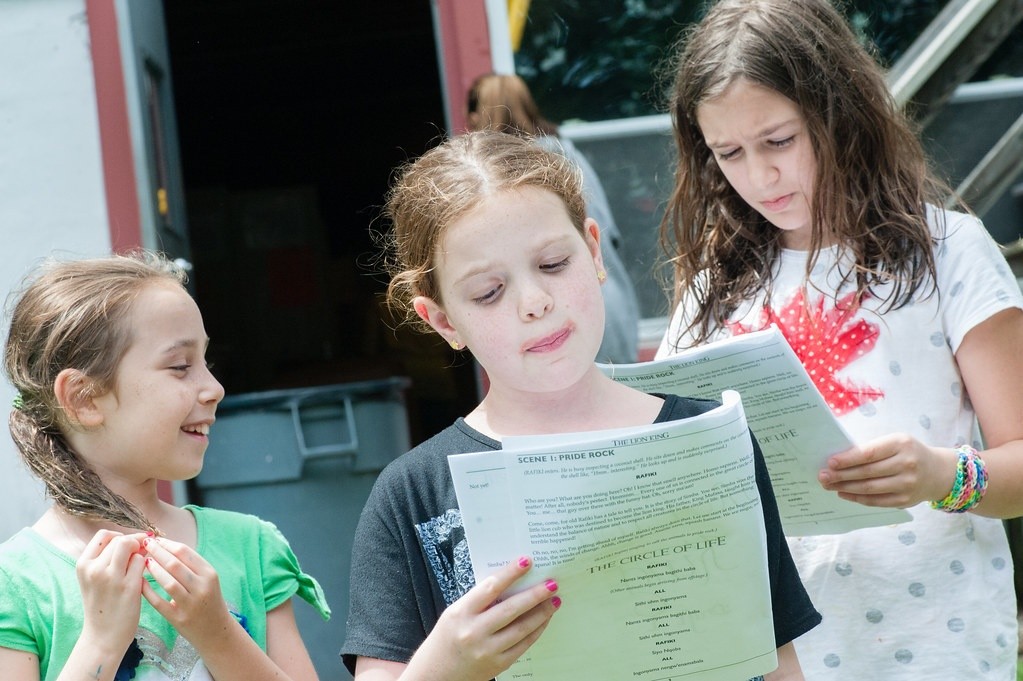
198 374 414 681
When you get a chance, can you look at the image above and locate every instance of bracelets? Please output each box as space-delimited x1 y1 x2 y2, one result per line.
929 445 989 513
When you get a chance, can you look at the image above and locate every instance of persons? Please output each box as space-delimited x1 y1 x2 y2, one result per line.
0 256 332 681
466 72 639 364
338 131 823 681
656 0 1023 681
